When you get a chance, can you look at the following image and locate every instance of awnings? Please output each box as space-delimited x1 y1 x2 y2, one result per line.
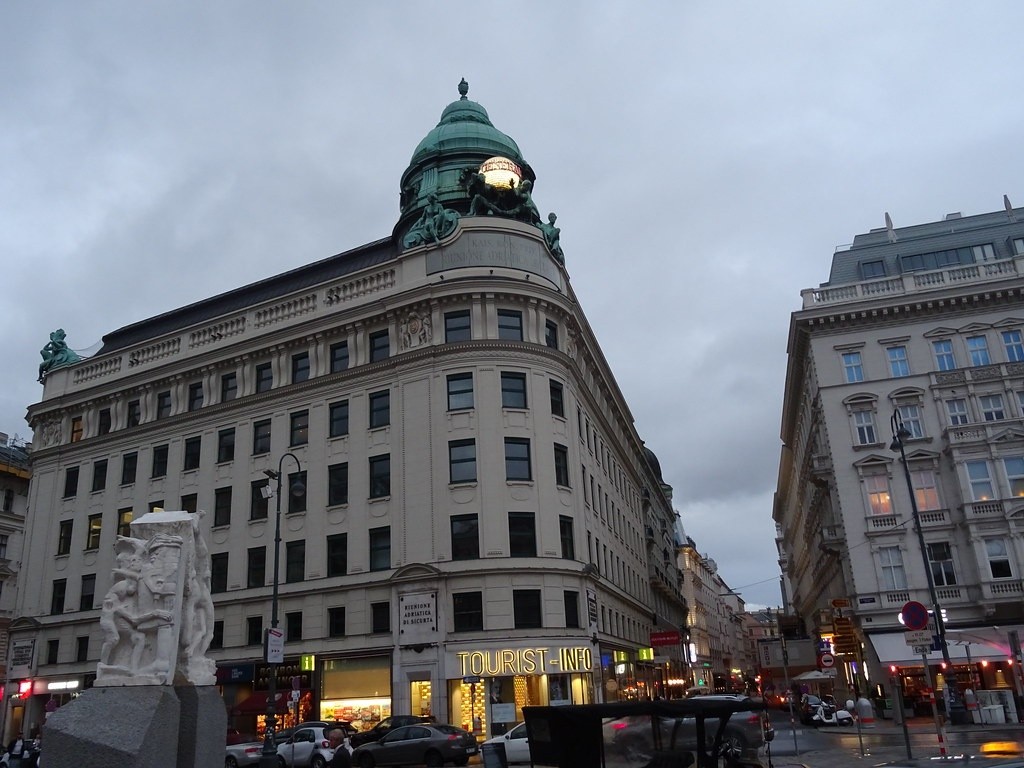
869 624 1024 668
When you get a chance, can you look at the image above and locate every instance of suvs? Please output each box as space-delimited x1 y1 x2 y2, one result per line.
621 692 776 763
273 720 359 748
349 715 439 747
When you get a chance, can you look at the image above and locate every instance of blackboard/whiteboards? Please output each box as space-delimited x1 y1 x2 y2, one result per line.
491 703 516 723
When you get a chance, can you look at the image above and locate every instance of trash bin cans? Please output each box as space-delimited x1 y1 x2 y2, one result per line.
856 698 875 728
479 742 508 768
964 689 976 710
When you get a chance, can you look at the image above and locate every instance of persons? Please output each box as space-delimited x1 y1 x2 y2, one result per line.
550 675 564 699
489 680 508 736
0 732 42 768
690 689 744 698
101 510 215 674
763 686 771 707
667 689 671 700
399 318 411 351
799 689 808 725
329 729 351 768
536 213 566 269
419 312 430 345
646 694 666 721
466 178 533 221
418 193 446 246
37 329 67 381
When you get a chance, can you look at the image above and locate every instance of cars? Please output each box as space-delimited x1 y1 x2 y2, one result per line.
797 691 839 726
477 717 631 764
226 729 257 746
779 694 796 709
272 726 354 768
353 722 480 768
224 741 264 768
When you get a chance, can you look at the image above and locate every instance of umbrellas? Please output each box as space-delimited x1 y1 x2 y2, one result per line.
686 684 709 692
792 670 834 698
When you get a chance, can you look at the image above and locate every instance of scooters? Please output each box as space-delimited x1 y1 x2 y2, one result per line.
809 701 854 729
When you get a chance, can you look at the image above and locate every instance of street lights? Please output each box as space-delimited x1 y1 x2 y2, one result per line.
885 406 972 725
259 452 307 768
